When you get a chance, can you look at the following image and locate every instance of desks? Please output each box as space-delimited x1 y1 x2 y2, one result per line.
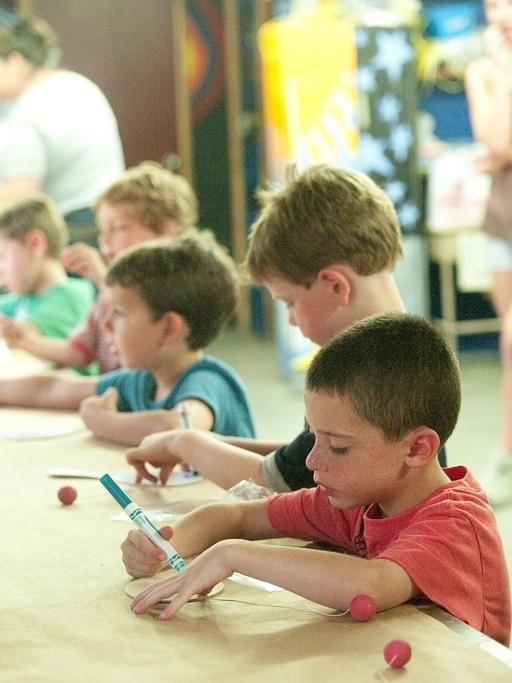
0 348 512 683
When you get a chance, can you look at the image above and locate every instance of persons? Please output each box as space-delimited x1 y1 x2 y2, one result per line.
1 189 97 377
465 0 512 450
0 238 255 446
1 13 123 280
125 161 448 499
2 160 199 372
119 310 511 647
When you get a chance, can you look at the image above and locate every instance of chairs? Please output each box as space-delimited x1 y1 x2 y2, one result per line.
428 141 503 362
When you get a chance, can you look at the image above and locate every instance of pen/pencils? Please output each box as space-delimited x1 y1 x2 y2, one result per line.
178 401 198 476
99 473 186 574
